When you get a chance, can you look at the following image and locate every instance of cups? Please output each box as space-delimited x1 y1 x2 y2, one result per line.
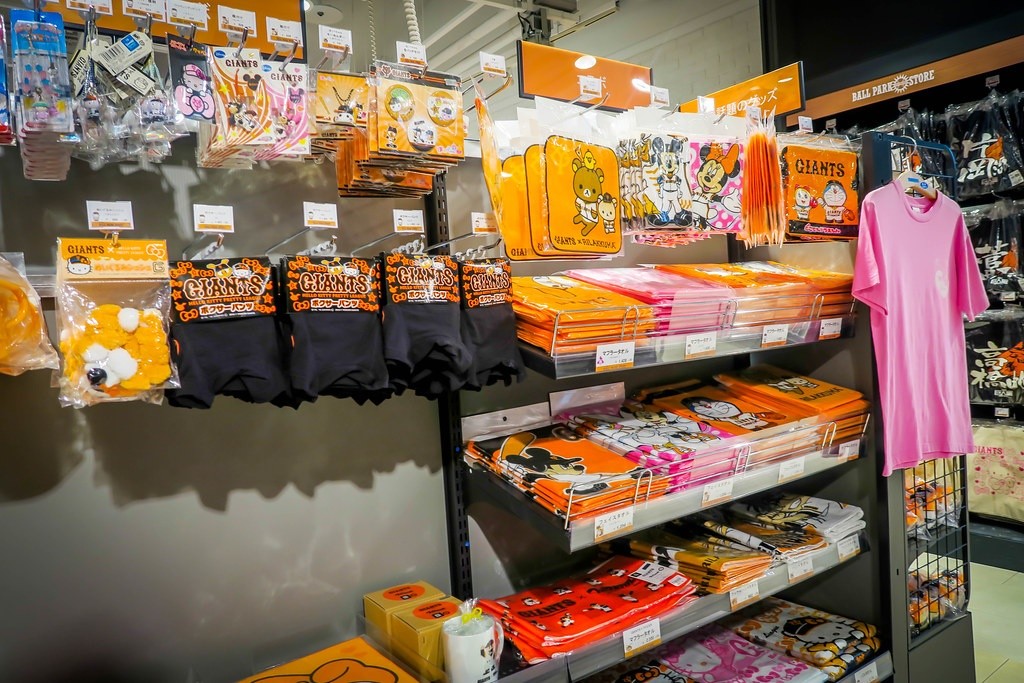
442 615 505 683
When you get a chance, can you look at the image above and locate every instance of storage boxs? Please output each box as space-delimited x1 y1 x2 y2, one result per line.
364 578 466 681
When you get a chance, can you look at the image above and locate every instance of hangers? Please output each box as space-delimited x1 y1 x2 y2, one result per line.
895 134 937 202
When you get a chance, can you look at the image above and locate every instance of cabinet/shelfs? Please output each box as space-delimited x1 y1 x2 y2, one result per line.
427 88 893 683
